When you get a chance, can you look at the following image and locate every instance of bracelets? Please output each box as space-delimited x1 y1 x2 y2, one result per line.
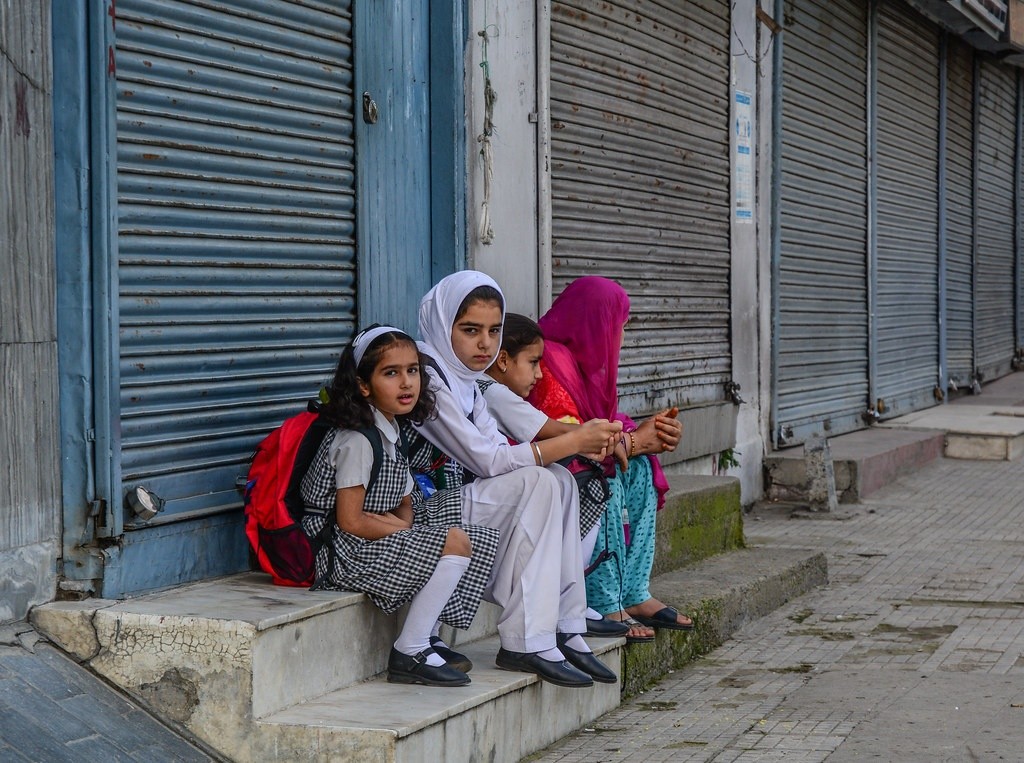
629 431 636 456
533 441 544 466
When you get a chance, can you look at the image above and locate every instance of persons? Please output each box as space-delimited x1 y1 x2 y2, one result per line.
393 270 624 687
298 322 502 686
462 311 630 636
506 276 696 644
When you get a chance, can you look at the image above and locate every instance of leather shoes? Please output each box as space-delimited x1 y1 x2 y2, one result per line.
580 608 630 637
387 644 472 686
557 634 617 683
430 636 474 673
495 647 596 687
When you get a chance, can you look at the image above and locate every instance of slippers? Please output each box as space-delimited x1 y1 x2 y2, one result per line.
619 618 656 643
630 605 696 630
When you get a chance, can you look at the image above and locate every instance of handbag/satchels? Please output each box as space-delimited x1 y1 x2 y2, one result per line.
555 454 610 504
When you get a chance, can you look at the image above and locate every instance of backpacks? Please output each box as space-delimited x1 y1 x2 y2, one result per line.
242 401 384 590
